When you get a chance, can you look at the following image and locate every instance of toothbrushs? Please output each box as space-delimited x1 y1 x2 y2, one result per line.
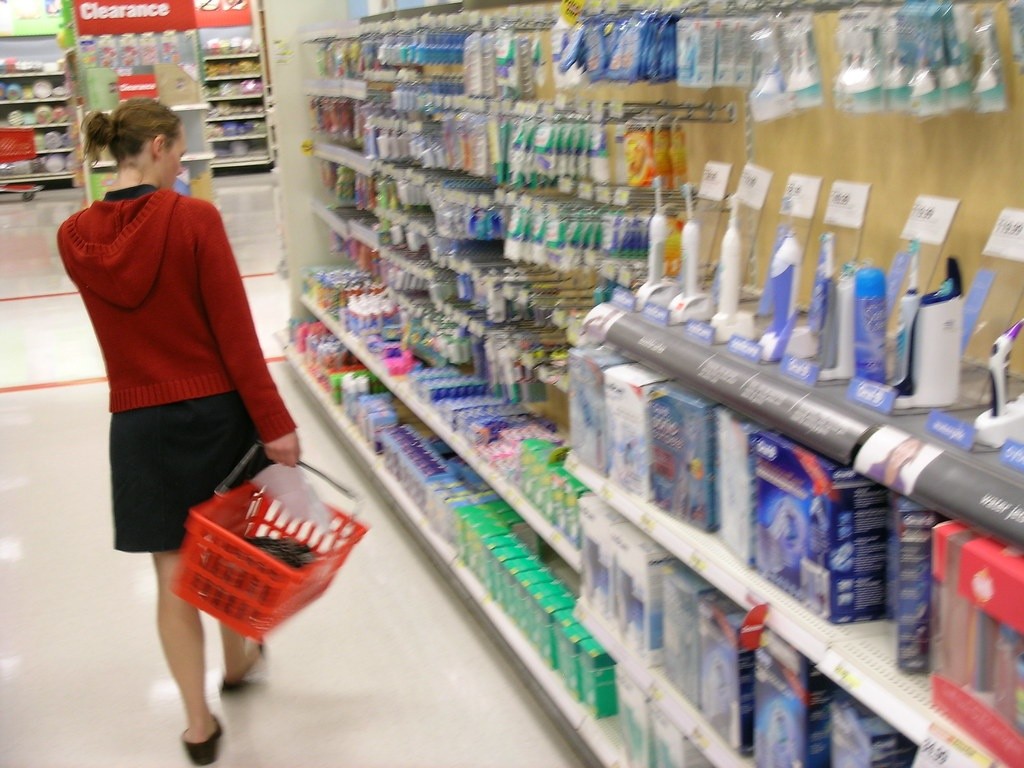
646 175 1024 418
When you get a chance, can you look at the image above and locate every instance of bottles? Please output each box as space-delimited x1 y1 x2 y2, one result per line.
339 291 400 337
331 366 387 412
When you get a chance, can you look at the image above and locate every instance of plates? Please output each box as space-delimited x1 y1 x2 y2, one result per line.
0 58 64 73
0 81 66 100
14 155 77 175
36 132 71 149
8 105 68 126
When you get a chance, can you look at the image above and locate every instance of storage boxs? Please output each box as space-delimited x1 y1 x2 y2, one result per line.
355 341 1024 768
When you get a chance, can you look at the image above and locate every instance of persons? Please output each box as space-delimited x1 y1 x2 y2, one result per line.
58 99 301 764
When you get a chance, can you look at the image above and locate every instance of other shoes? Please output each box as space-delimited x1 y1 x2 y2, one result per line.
180 714 222 765
222 645 262 692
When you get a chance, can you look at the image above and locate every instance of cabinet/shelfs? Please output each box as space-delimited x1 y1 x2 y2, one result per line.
569 304 1024 767
276 0 619 768
0 0 276 213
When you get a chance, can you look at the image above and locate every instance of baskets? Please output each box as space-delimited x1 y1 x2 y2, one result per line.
168 440 369 641
